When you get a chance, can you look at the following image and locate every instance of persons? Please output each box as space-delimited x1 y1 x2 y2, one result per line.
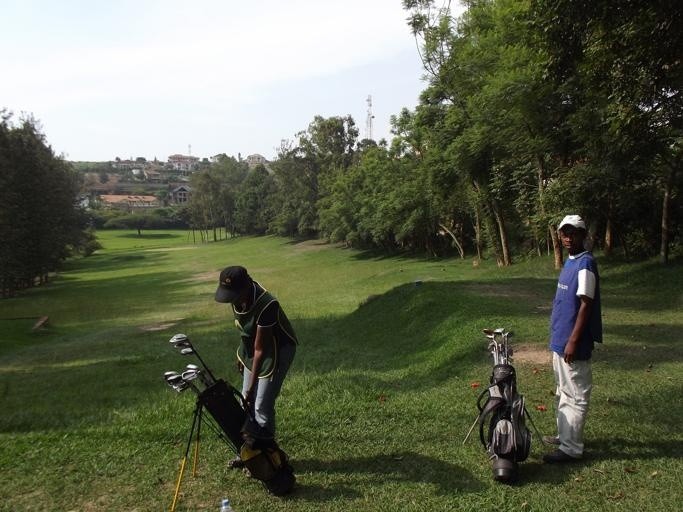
540 213 605 463
214 264 299 478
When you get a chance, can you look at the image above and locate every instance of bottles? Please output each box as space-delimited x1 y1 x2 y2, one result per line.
220 499 235 512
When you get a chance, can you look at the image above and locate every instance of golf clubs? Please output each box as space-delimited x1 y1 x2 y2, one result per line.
164 333 218 396
483 328 513 366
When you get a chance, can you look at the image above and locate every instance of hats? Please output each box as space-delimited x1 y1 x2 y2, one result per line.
214 266 248 303
558 214 586 230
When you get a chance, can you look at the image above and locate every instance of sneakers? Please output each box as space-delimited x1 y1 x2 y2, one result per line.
542 435 560 444
227 458 243 469
544 448 571 463
